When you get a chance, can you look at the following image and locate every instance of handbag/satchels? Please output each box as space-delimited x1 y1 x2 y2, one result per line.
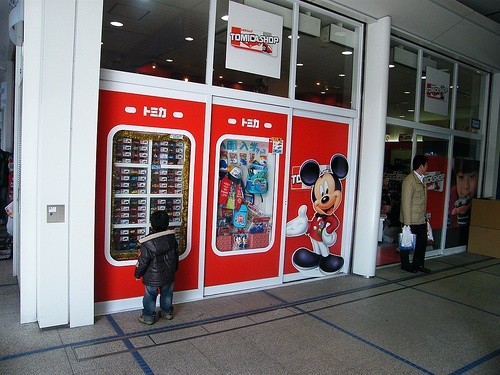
399 225 417 251
427 221 433 245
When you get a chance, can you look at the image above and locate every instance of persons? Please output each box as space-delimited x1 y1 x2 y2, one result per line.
134 210 178 324
447 159 479 228
399 154 432 273
385 192 401 237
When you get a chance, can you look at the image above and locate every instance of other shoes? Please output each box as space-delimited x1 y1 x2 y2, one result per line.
401 265 418 273
161 311 173 320
418 265 431 273
137 315 154 325
